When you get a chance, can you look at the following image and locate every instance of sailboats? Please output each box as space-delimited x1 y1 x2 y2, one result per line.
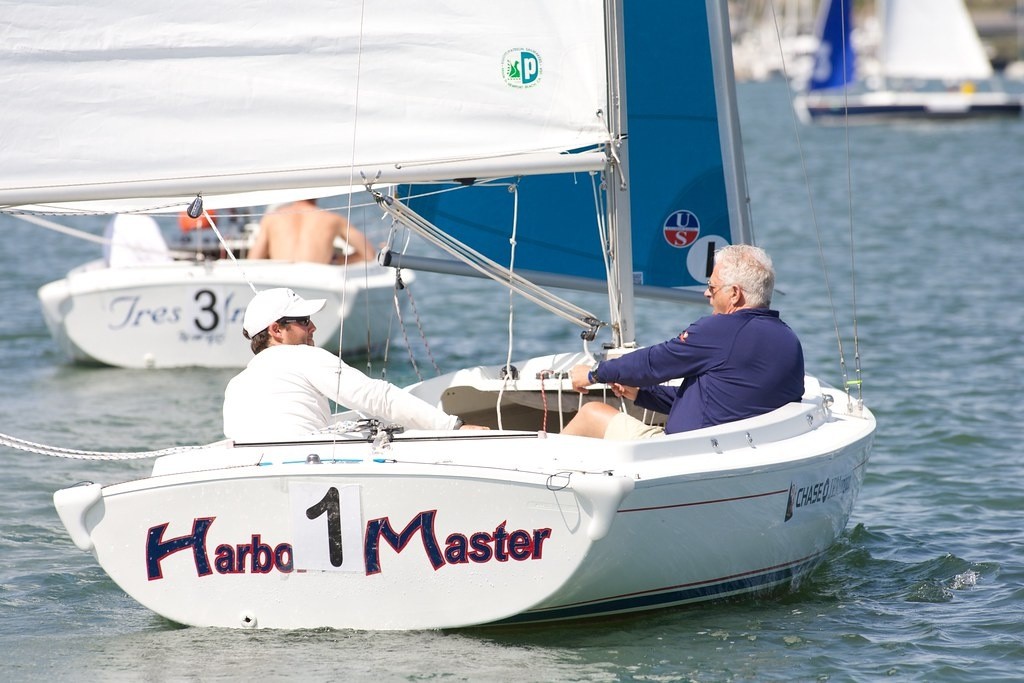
790 0 1024 127
0 183 417 369
1 0 878 632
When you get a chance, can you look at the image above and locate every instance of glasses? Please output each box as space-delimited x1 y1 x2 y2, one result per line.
284 316 312 327
706 279 752 298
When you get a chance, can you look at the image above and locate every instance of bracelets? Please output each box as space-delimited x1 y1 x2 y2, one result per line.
588 372 596 384
453 418 465 430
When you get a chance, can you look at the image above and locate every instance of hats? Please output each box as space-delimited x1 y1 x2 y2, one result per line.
243 288 328 339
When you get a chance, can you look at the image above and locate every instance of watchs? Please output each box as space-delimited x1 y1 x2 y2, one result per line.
590 360 601 382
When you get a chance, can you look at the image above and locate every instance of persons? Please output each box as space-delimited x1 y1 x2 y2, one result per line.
246 197 374 264
557 243 805 439
221 287 491 443
